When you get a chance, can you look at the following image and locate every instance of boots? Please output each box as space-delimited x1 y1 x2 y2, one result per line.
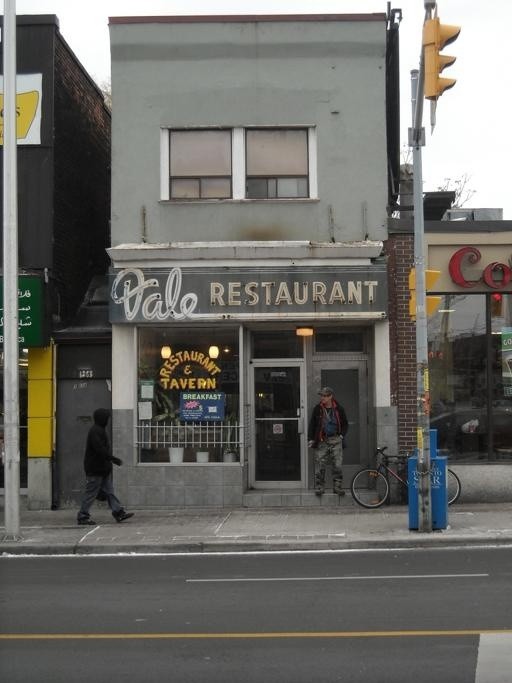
76 510 97 525
333 479 345 496
314 483 326 496
111 506 135 523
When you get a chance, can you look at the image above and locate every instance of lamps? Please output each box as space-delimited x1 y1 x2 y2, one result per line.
296 326 313 336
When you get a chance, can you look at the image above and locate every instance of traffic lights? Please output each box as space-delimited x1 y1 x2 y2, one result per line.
420 14 462 103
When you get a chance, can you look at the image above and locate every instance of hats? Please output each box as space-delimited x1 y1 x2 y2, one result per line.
318 386 334 396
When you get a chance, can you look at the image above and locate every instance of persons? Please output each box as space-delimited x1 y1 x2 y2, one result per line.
307 385 348 496
76 407 135 525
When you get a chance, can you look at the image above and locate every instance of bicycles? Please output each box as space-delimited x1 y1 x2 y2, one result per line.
349 444 462 508
